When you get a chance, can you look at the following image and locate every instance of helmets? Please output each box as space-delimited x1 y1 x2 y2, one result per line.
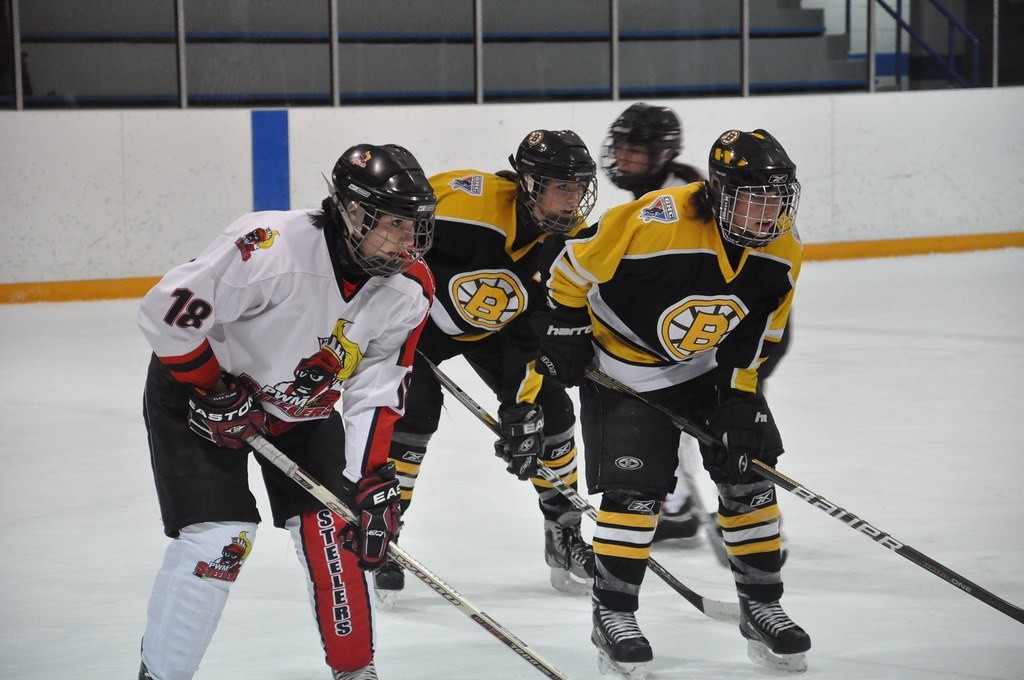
507 130 598 233
322 144 438 279
599 103 682 190
708 130 799 248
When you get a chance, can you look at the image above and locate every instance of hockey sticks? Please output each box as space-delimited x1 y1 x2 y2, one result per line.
585 365 1024 625
244 431 571 680
416 346 743 620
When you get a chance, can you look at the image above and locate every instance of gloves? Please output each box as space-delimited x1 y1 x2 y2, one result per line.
188 373 267 450
494 403 545 481
339 461 399 569
706 397 765 479
533 315 596 388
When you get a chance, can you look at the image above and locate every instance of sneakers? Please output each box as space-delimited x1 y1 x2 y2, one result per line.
331 658 378 680
740 595 812 671
651 498 704 550
370 556 405 609
590 598 654 680
544 521 596 595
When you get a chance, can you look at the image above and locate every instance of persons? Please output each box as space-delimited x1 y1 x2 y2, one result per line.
597 101 731 550
535 129 811 680
134 141 438 680
376 128 599 611
